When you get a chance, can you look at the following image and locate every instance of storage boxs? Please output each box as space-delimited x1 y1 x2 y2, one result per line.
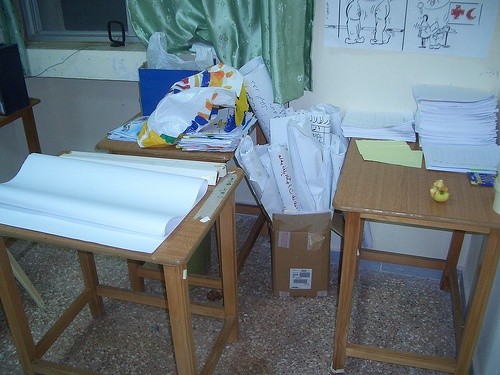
138 59 217 118
270 212 345 297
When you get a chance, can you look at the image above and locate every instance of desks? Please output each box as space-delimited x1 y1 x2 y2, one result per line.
332 136 500 375
0 98 42 155
97 110 266 293
0 151 242 375
